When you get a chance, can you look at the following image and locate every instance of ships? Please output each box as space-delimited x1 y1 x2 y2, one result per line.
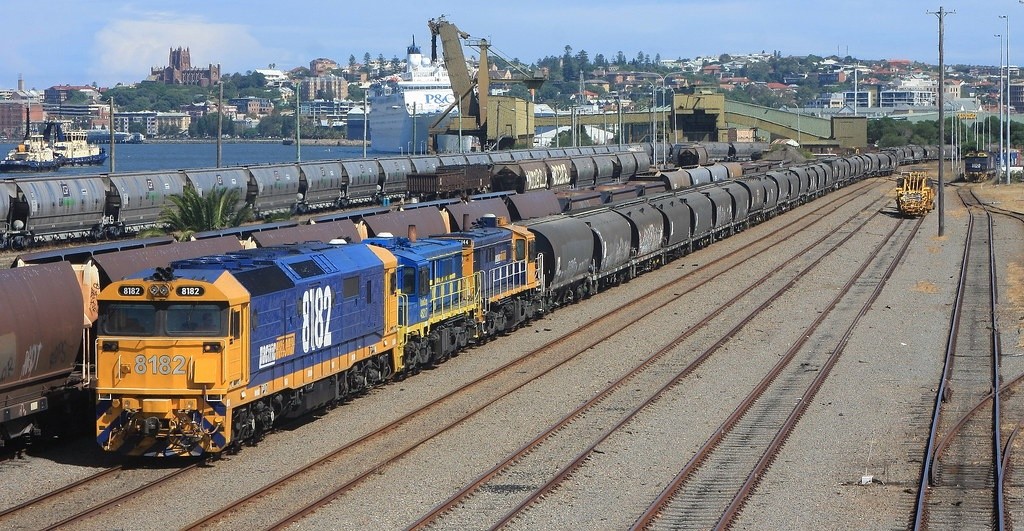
368 34 478 153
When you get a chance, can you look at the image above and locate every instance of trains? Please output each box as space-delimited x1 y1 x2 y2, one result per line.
0 144 997 469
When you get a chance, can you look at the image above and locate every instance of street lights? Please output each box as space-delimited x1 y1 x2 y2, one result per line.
998 14 1012 187
994 33 1003 184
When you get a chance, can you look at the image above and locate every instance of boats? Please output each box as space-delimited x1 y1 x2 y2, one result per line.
0 106 110 172
282 137 294 145
